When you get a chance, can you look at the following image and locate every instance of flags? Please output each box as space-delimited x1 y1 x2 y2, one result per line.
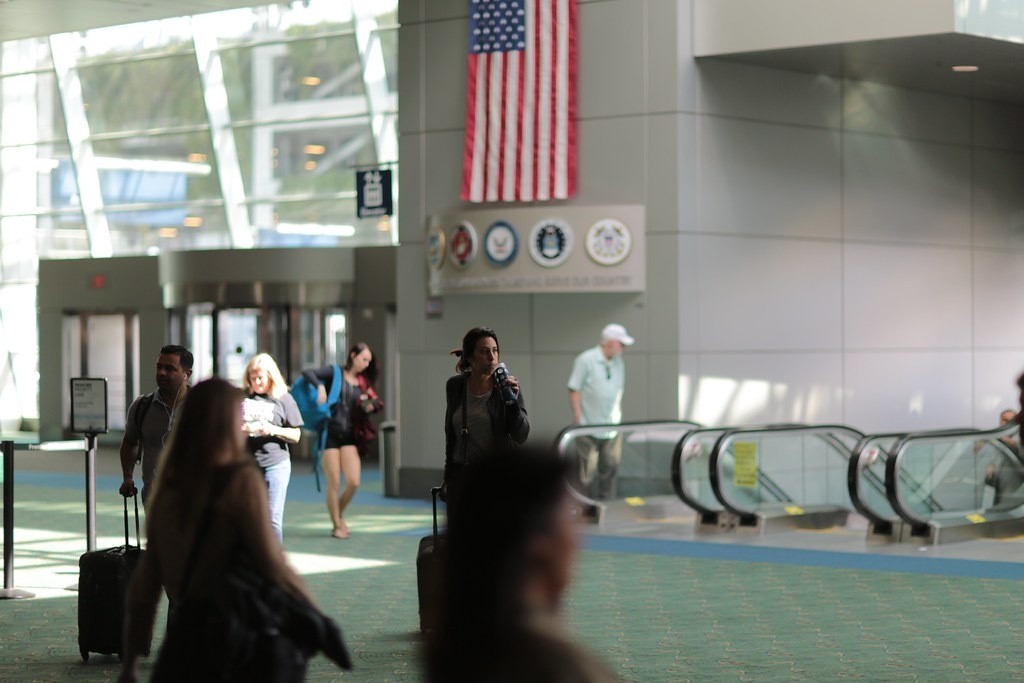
460 0 578 203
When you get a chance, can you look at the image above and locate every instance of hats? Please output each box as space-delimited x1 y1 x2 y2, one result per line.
602 323 635 346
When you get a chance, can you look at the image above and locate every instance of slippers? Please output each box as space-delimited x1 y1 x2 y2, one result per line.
333 525 349 538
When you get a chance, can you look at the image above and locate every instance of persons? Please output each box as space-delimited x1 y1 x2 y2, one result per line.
116 379 321 683
118 343 193 516
567 323 635 517
980 408 1020 507
438 326 532 502
302 342 379 539
240 352 305 541
418 443 610 683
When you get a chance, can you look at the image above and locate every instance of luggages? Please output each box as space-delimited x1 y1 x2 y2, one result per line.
415 487 455 634
78 489 154 661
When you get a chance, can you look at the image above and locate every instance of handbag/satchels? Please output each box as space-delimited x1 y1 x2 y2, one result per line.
148 460 307 683
289 365 342 430
446 462 466 511
